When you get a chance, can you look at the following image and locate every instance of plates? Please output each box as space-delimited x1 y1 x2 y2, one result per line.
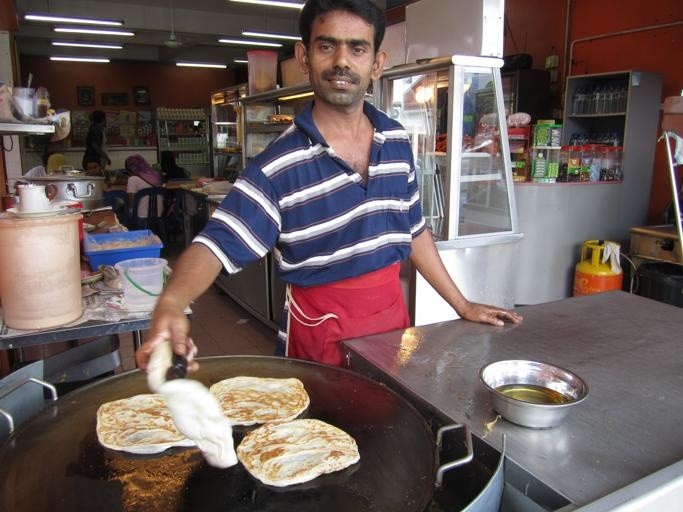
81 273 103 284
7 207 66 216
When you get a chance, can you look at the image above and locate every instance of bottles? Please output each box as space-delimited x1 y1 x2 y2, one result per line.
570 132 621 147
558 144 571 182
163 135 167 148
574 88 626 113
160 136 164 148
178 137 202 144
178 153 207 163
590 144 601 181
14 89 37 119
611 147 623 180
580 144 591 182
601 146 614 181
568 145 582 182
156 105 205 121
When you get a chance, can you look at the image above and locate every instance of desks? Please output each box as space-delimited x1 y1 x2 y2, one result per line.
1 254 193 373
101 181 186 192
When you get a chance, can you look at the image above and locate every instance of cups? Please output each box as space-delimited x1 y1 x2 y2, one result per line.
17 184 50 208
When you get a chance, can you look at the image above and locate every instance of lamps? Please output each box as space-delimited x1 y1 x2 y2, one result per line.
240 31 304 41
217 38 286 48
50 41 124 50
233 58 249 64
22 14 123 26
164 22 183 50
402 73 436 105
47 56 111 64
53 28 136 36
175 63 230 69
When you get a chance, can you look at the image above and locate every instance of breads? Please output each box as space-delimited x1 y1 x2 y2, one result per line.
266 113 294 123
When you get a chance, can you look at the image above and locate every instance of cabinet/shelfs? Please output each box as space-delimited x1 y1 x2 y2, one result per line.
559 71 630 183
502 68 552 123
209 102 244 175
245 82 316 173
203 198 289 324
156 105 214 179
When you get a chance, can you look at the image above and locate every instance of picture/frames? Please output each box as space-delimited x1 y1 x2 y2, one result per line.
132 84 151 106
101 93 129 106
76 85 95 107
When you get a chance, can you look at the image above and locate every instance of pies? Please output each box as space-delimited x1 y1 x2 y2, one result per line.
237 418 360 487
208 376 310 426
158 378 239 470
96 393 197 456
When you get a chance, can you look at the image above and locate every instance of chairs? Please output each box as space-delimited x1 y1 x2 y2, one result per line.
101 186 185 260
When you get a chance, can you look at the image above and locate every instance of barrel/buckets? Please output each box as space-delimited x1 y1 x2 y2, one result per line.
115 257 167 310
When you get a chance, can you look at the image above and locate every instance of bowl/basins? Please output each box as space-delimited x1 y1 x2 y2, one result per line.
481 359 590 427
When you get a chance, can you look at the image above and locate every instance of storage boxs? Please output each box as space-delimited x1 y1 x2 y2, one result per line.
238 83 248 99
209 89 224 104
82 229 166 274
225 85 238 101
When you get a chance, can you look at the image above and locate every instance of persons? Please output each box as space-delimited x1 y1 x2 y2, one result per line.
125 154 165 226
134 0 525 376
82 110 112 175
21 142 65 185
159 151 192 247
